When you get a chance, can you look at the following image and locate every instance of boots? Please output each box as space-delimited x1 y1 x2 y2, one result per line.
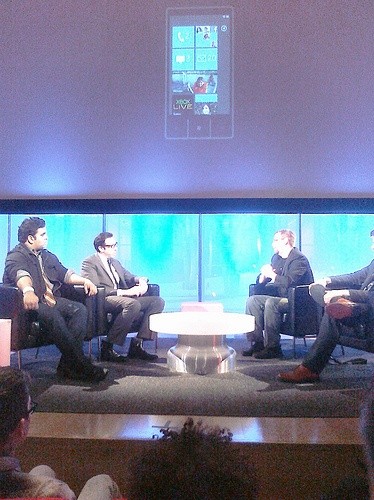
128 337 158 360
101 337 128 361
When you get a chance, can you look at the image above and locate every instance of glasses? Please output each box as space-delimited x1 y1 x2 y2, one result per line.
27 402 37 414
105 242 118 249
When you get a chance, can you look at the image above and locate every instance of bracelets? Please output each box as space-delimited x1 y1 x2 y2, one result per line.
23 287 34 293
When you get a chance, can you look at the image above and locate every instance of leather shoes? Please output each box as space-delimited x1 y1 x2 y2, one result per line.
276 365 320 383
324 298 361 320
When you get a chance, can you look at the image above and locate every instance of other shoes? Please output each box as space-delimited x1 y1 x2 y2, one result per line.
252 350 284 359
57 369 80 380
242 345 265 356
78 363 108 382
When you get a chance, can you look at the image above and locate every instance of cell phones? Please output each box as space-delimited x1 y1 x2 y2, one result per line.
165 5 233 141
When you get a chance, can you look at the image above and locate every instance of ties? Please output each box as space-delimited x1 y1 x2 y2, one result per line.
107 260 119 289
36 255 56 308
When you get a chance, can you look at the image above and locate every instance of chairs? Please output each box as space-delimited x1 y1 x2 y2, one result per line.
249 274 374 360
0 279 160 369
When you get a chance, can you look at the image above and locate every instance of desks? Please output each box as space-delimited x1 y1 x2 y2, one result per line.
148 312 256 377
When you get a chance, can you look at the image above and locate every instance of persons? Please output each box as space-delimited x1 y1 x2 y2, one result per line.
4 218 110 380
0 365 121 500
242 229 314 358
278 230 374 383
194 77 206 92
81 232 165 361
